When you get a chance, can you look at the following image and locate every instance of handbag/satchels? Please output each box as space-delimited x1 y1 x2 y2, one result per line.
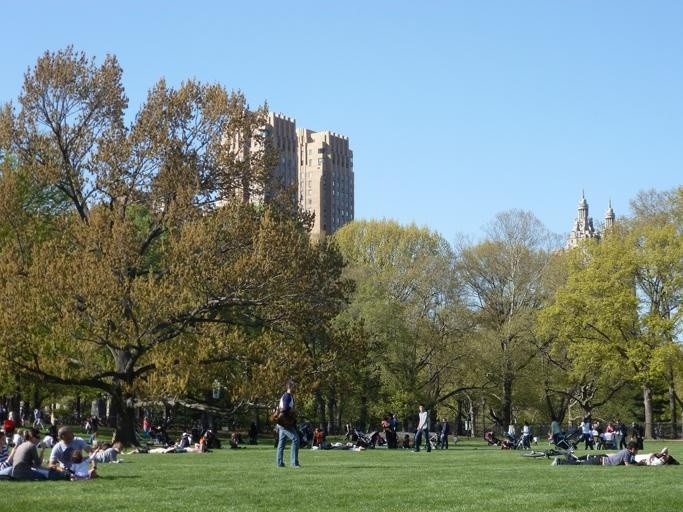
270 407 299 425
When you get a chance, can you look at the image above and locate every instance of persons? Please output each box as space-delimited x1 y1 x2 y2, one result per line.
306 402 452 452
130 412 259 457
0 397 130 479
483 411 681 466
269 378 302 468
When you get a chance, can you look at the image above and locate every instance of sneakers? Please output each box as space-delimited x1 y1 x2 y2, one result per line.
551 457 558 466
